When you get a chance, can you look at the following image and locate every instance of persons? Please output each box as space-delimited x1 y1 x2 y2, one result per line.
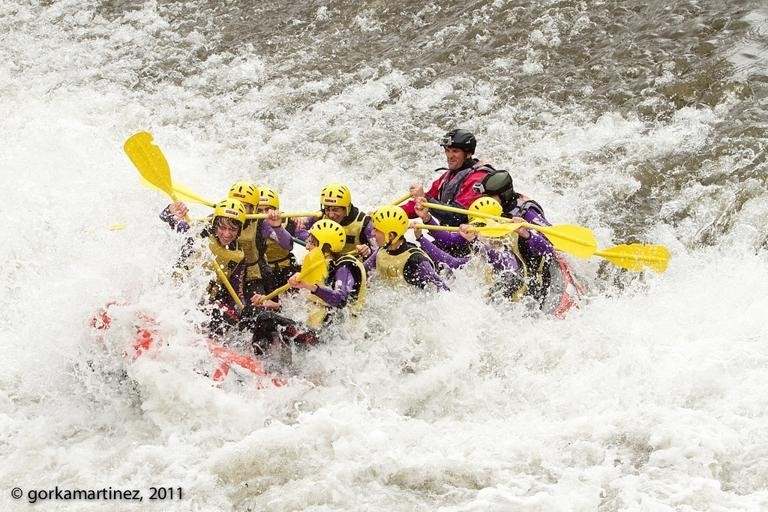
158 128 555 359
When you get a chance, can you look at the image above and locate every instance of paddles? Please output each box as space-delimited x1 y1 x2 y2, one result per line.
124 131 245 314
249 248 328 306
407 224 524 237
419 200 597 260
554 243 670 275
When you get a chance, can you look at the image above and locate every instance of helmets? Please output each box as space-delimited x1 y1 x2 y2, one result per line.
482 169 513 202
309 218 346 252
441 129 477 152
468 197 504 225
320 184 352 217
212 180 279 237
371 205 409 245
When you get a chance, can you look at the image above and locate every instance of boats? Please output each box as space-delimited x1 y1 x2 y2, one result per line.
90 263 584 412
